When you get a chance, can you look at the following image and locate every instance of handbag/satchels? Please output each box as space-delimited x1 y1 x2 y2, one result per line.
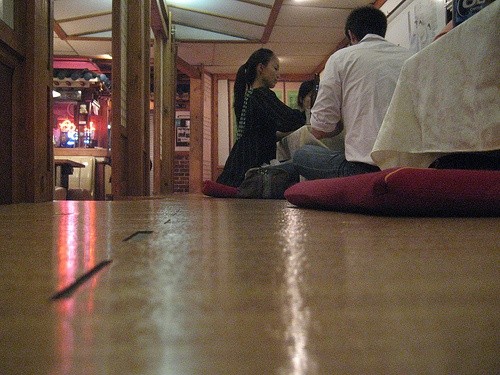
234 166 290 199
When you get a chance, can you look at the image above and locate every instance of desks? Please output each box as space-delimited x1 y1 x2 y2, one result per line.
370 0 500 169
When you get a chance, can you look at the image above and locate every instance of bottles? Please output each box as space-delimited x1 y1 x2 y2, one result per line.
310 73 320 116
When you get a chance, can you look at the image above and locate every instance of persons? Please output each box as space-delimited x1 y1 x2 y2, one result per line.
215 48 307 187
292 6 429 187
